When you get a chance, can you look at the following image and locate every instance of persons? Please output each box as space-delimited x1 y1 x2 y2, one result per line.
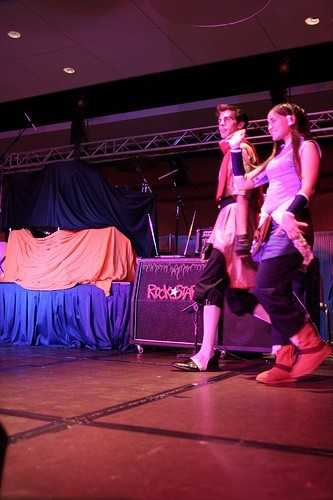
230 104 331 384
172 105 264 371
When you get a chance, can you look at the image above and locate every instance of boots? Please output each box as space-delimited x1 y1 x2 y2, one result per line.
256 344 300 384
288 322 332 377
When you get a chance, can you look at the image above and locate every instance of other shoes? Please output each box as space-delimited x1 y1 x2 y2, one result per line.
172 353 220 372
